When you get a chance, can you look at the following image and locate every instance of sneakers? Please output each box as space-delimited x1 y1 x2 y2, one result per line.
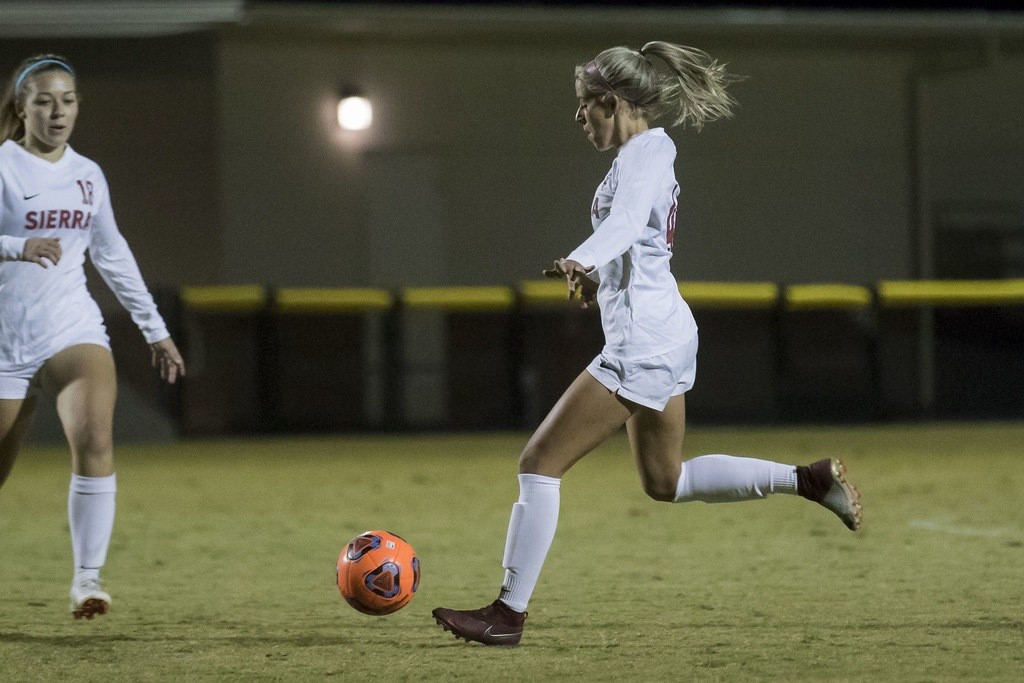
68 577 113 621
796 453 864 534
432 597 528 647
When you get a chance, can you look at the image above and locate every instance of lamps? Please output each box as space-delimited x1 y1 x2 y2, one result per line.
337 85 372 129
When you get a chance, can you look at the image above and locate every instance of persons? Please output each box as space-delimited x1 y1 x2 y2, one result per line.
431 41 864 648
0 54 185 619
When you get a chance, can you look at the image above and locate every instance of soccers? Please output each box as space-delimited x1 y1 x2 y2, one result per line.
334 529 422 618
